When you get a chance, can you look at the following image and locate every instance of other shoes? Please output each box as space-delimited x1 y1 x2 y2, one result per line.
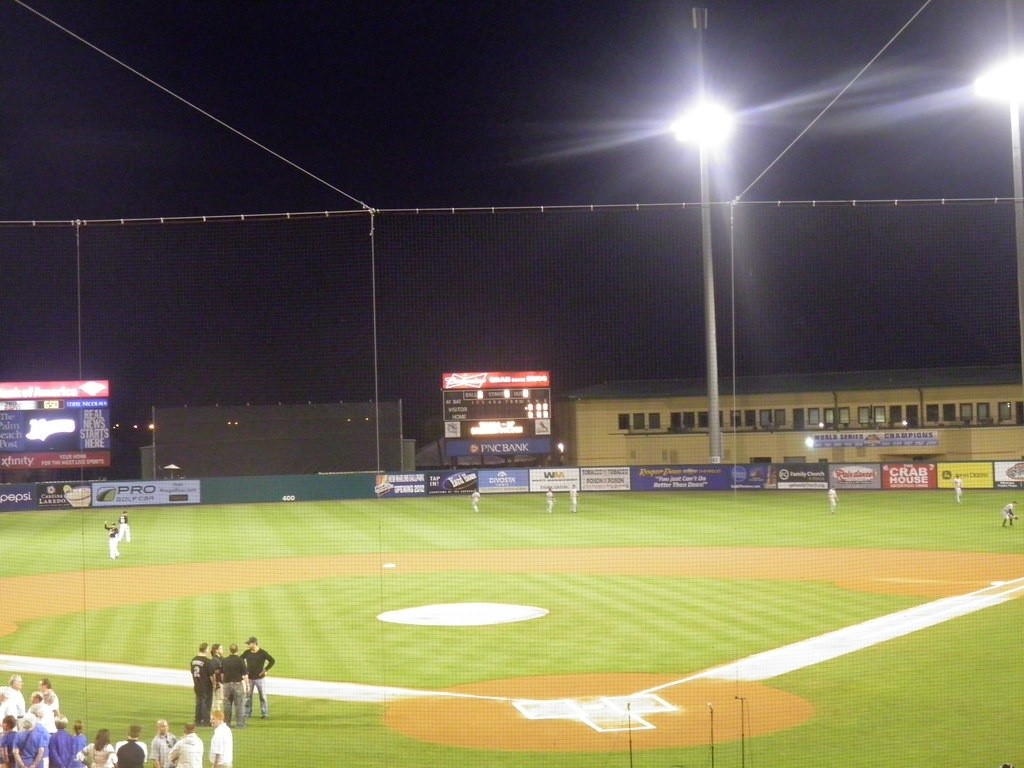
194 720 213 726
237 724 249 729
245 712 252 717
262 715 268 719
227 722 232 727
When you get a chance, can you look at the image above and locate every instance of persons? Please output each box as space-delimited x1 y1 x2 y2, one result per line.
115 725 148 768
105 520 120 559
168 723 204 768
570 484 578 513
150 720 177 768
221 643 248 728
1001 501 1017 528
209 710 233 768
0 675 60 736
471 489 480 513
953 476 963 503
240 636 274 719
827 486 838 512
0 714 88 768
118 511 131 543
546 487 556 513
76 729 118 768
190 643 224 728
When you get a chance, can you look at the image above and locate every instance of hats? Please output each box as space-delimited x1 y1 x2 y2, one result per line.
111 522 116 525
245 637 257 644
